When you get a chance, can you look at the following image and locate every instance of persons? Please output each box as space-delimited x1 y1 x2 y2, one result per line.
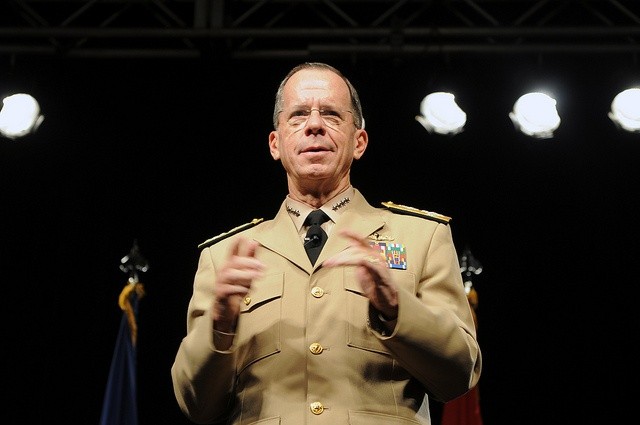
172 63 482 424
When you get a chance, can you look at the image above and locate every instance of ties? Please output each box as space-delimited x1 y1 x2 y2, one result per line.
304 210 331 268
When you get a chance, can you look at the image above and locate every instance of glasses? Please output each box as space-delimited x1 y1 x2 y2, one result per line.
274 104 360 126
